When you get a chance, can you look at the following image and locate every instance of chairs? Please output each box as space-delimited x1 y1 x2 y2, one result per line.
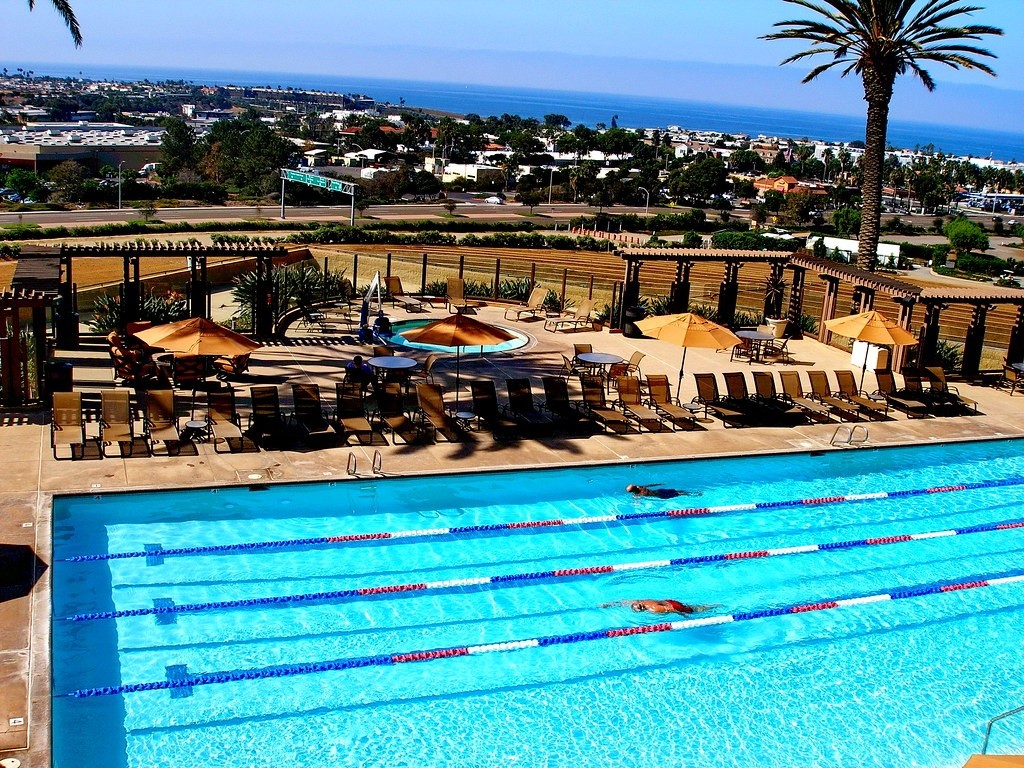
51 275 1024 461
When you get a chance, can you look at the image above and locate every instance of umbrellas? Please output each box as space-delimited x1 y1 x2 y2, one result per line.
133 317 261 421
399 314 519 402
633 313 743 407
824 310 919 396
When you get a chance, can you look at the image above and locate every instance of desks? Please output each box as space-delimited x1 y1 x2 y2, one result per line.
577 352 624 381
184 420 207 444
1012 363 1024 372
368 356 418 383
735 330 775 363
455 411 475 431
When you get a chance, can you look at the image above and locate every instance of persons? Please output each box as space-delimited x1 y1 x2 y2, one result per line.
626 484 664 496
347 356 374 386
632 599 686 614
375 310 392 334
